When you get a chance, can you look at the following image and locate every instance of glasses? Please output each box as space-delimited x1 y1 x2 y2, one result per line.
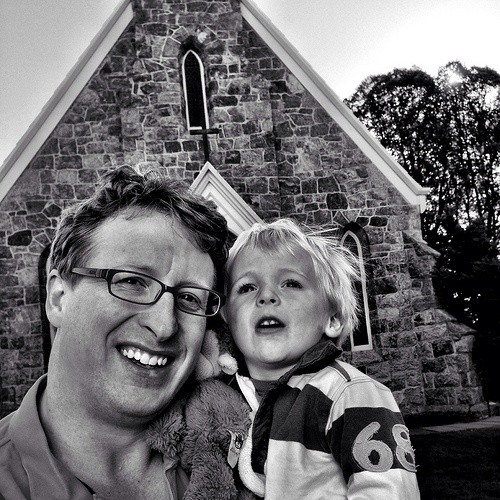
68 265 222 319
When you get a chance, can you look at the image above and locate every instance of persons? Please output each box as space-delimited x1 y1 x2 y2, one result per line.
0 170 263 500
221 219 421 500
